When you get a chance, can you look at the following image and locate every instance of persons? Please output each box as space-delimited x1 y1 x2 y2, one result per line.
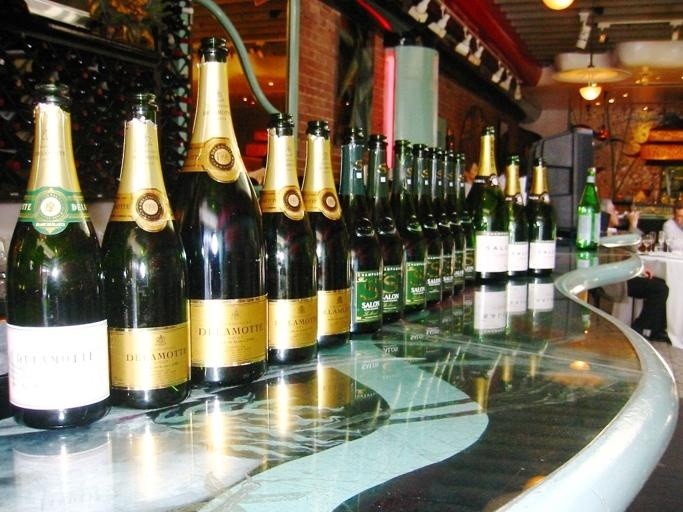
658 199 683 250
591 196 669 345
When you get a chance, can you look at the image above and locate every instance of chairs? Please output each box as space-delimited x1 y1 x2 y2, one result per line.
592 292 638 327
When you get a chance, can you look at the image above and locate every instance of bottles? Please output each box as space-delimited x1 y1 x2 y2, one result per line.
301 120 353 350
338 125 475 332
258 109 314 365
179 35 270 388
3 82 110 429
98 93 199 409
471 277 557 416
573 164 600 252
8 434 115 511
468 128 559 287
572 249 602 338
348 288 592 439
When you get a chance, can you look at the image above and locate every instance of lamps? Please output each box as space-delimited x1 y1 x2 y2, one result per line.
552 6 683 100
543 0 574 10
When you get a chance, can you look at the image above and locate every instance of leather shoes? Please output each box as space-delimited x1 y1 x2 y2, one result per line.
650 330 669 340
